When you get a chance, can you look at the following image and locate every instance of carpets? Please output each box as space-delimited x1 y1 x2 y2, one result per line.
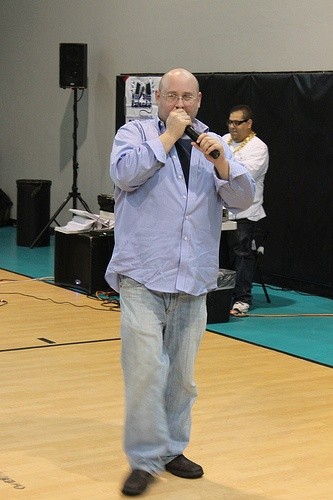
0 223 333 368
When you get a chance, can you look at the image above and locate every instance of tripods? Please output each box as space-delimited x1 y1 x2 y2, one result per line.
29 89 91 249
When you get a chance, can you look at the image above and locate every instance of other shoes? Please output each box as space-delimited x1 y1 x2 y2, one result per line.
232 301 249 313
121 469 149 496
164 454 204 479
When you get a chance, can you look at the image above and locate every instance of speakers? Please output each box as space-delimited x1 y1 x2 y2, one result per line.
59 42 87 90
54 232 116 293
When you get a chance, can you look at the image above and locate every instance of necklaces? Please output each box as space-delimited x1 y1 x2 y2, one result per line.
228 131 256 153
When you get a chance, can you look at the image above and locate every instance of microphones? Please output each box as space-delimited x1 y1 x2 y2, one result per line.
185 125 220 159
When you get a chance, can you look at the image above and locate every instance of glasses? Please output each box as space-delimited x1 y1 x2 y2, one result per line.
160 93 198 102
226 119 249 126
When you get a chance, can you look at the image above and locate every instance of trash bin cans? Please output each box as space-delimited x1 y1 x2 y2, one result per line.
16 179 52 246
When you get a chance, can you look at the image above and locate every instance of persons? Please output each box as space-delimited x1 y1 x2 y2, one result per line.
221 105 269 312
104 68 257 496
